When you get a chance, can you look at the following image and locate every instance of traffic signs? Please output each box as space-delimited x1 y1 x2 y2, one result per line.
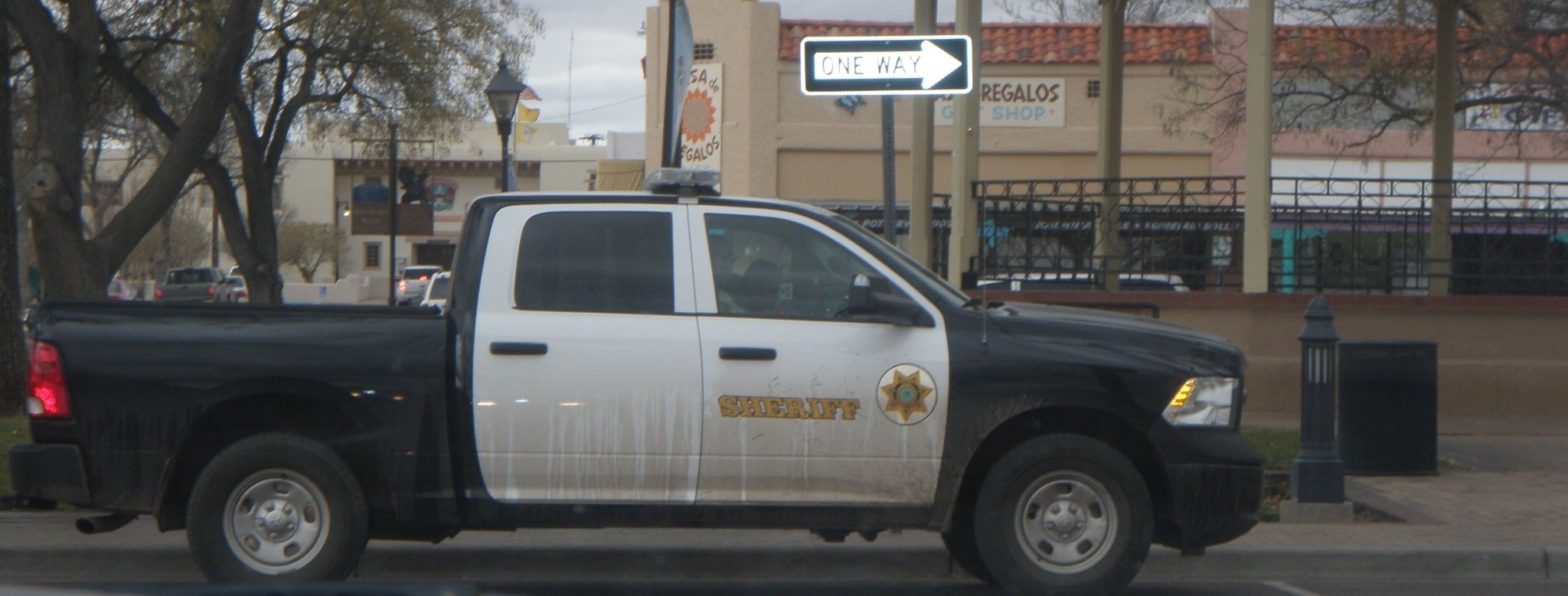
800 34 973 95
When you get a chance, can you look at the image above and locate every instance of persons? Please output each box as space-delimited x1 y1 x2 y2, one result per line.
708 234 824 319
107 276 124 300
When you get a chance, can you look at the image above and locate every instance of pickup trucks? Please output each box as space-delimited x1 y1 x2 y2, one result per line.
10 191 1264 596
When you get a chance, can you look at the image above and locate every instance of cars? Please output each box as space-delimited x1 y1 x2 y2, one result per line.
977 273 1187 296
393 266 443 303
106 280 126 300
214 265 250 304
154 267 221 300
414 271 452 312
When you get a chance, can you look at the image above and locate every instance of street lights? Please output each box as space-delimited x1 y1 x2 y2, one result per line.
483 53 526 193
